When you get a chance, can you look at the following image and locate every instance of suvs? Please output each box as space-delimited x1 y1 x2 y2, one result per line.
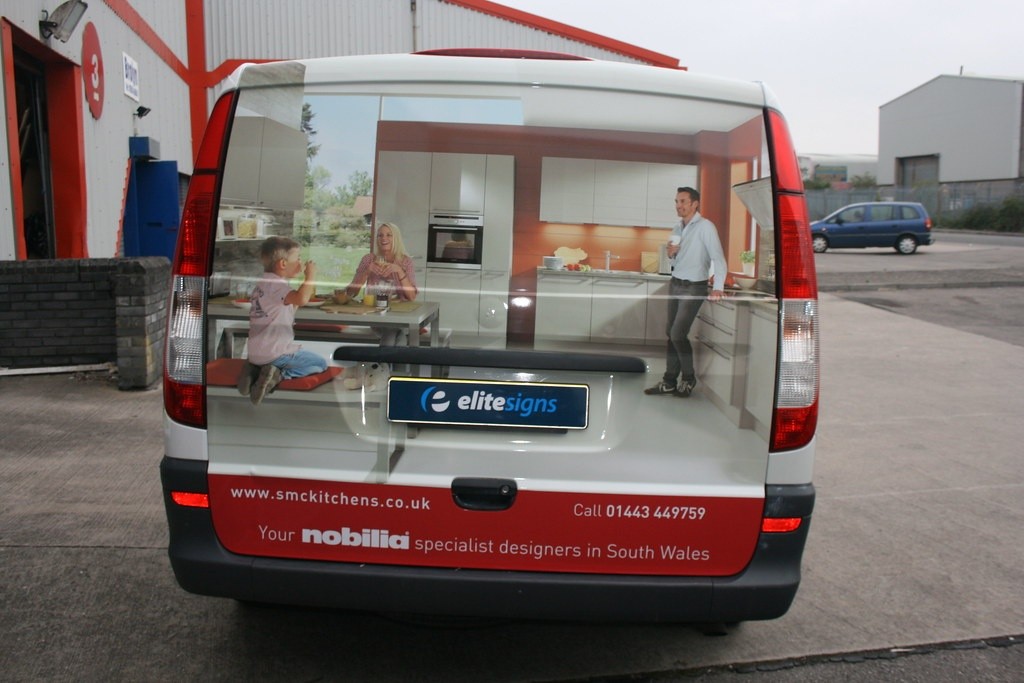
809 201 934 255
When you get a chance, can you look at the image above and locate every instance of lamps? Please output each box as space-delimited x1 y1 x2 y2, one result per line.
132 105 151 118
38 0 88 45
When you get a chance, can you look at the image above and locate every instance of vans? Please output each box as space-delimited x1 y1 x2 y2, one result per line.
161 53 822 630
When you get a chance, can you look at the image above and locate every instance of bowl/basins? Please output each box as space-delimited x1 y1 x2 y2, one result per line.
735 276 757 289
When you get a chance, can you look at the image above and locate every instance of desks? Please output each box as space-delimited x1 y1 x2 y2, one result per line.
207 298 451 439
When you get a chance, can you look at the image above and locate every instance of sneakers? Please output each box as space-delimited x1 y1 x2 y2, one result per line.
250 364 282 405
645 381 676 396
677 375 697 395
237 358 257 395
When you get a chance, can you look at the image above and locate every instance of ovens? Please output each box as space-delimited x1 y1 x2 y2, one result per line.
426 209 485 270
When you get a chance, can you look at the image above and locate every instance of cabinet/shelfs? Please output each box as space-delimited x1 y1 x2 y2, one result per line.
372 152 777 442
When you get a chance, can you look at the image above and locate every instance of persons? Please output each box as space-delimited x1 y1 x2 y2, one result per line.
238 237 327 405
645 188 728 397
335 221 418 390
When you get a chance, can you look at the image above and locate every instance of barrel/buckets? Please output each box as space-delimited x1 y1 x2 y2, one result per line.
641 251 660 273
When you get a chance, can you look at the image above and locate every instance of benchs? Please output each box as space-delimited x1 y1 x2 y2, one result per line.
205 356 421 477
222 322 455 380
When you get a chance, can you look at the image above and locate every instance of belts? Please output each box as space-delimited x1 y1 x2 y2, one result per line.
672 276 707 285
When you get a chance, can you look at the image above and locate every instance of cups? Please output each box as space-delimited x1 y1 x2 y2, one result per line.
669 235 681 247
301 261 322 279
237 283 248 300
363 288 375 307
335 288 348 304
376 255 386 272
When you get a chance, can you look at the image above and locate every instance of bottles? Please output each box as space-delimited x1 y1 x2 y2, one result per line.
237 213 257 238
377 288 389 307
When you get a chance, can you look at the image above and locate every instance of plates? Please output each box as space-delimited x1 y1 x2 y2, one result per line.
543 255 563 269
305 299 325 307
231 299 251 309
